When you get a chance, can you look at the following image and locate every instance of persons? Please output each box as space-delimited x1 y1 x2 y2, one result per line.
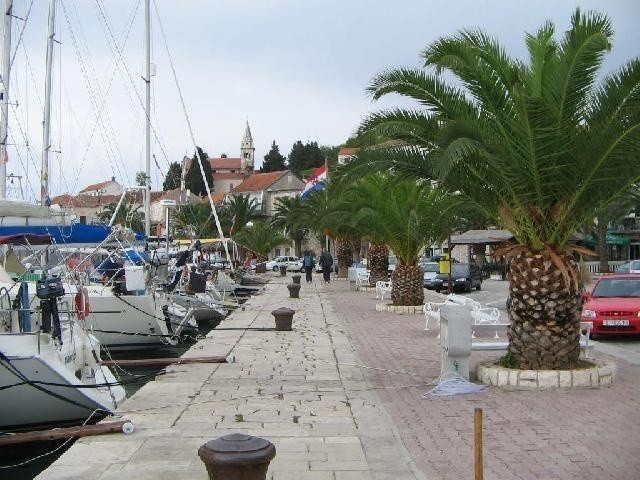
303 250 333 283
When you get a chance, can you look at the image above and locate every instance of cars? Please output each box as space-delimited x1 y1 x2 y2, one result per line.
387 255 397 278
580 260 640 340
423 261 483 294
266 256 338 273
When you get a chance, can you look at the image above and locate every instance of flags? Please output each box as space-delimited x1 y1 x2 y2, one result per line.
299 164 327 200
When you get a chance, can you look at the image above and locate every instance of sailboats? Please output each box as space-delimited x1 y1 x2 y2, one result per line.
0 0 243 432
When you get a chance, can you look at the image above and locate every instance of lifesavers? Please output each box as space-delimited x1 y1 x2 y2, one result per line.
75 288 89 320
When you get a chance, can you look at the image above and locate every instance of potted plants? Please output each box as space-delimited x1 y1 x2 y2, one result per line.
490 271 504 281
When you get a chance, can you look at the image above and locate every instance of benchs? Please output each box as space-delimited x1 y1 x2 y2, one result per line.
423 293 501 340
375 277 393 300
471 321 595 358
